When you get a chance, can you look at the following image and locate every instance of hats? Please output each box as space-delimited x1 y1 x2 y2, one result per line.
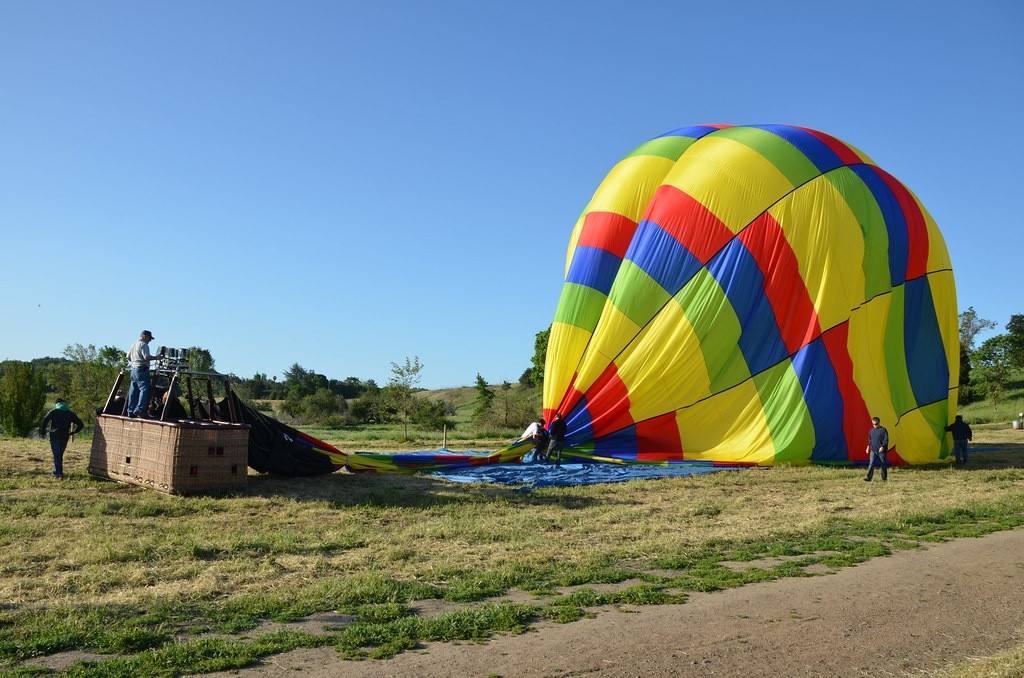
142 330 155 339
55 398 65 404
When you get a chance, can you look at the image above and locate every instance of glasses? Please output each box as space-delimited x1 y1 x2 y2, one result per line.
872 422 879 425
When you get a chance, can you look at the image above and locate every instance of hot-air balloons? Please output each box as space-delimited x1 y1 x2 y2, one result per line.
85 120 964 501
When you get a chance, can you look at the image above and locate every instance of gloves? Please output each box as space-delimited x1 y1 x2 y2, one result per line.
155 354 164 360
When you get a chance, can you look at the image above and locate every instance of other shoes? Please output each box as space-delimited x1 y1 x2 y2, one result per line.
130 415 136 418
133 410 150 419
53 470 65 478
863 478 871 481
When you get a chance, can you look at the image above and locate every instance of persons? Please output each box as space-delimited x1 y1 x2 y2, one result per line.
864 417 889 481
147 390 187 418
94 389 127 417
944 415 972 468
543 413 567 465
126 329 164 419
39 398 84 477
520 418 550 462
